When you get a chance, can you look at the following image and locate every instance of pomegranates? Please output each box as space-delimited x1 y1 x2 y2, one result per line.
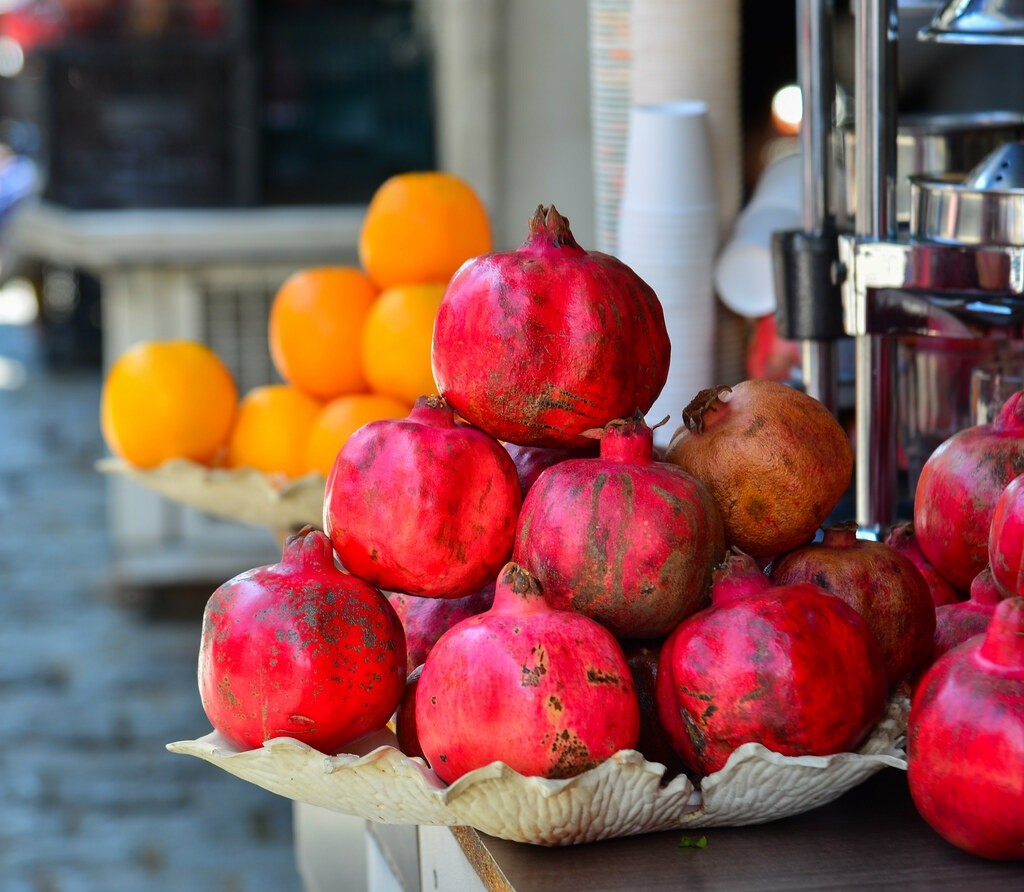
197 203 1023 866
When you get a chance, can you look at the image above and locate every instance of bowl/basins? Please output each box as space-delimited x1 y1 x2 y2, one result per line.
909 174 1024 245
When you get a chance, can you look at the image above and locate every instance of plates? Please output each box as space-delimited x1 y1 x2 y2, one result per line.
165 696 911 847
97 457 327 530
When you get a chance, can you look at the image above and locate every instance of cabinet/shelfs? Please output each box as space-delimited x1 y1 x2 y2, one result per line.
14 201 371 584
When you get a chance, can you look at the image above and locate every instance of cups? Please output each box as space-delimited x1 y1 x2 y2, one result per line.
589 0 805 448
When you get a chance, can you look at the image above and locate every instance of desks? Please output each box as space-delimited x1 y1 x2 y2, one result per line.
422 761 1024 892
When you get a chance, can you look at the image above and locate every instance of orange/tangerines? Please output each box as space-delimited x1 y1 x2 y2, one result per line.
100 169 490 483
